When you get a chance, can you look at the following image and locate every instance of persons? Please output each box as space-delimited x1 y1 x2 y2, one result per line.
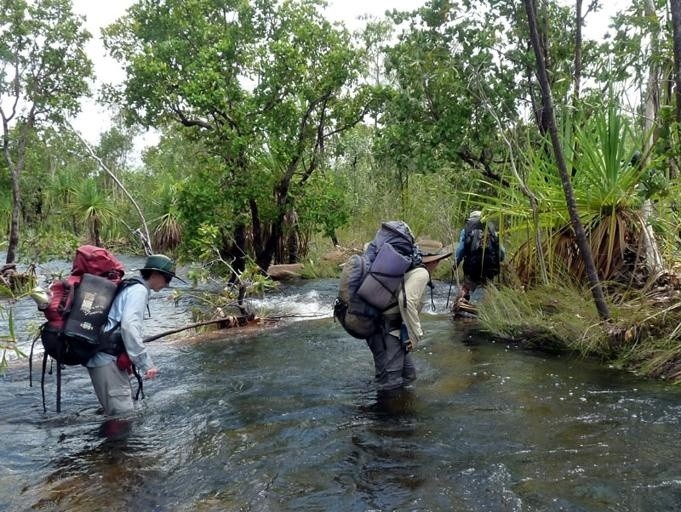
85 255 188 416
365 239 452 393
452 210 505 301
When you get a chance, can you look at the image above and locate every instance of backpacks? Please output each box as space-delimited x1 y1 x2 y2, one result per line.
335 220 416 339
38 244 152 370
463 221 498 283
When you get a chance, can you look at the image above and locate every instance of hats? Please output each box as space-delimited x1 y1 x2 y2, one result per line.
467 211 481 221
414 239 453 263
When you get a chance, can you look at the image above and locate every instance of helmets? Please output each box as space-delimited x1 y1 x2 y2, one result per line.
130 254 187 284
